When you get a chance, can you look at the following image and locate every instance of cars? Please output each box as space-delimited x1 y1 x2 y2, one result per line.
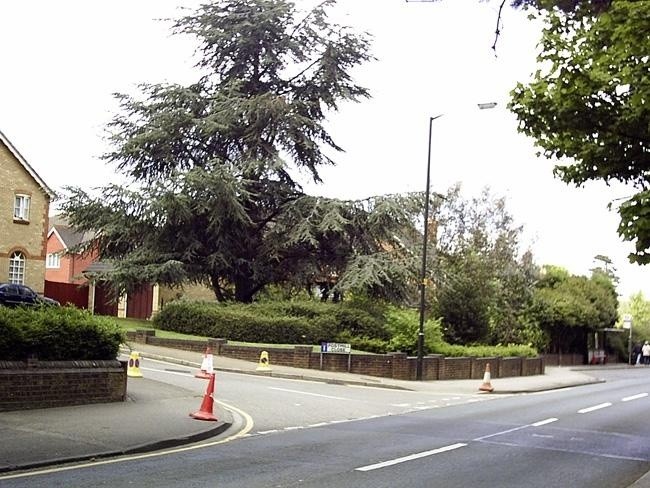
0 283 60 308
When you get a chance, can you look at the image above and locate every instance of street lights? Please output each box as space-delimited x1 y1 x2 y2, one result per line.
418 100 498 380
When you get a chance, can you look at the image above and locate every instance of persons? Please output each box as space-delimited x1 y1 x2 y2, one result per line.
641 340 650 366
632 339 643 366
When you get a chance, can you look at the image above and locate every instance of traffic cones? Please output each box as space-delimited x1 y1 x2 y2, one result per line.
195 344 215 379
189 373 219 420
478 362 495 391
